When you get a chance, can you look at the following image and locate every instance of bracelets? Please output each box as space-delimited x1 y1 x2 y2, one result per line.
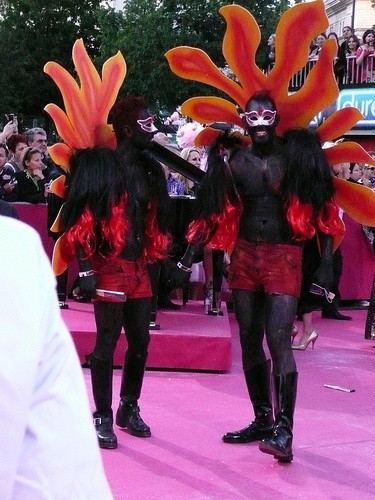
78 269 94 277
177 262 191 272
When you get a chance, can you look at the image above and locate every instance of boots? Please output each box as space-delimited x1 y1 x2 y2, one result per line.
89 353 117 449
115 348 151 437
260 371 298 463
223 358 275 444
360 301 375 340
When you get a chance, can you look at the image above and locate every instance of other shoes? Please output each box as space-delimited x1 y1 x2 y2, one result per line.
218 311 223 316
227 301 233 310
68 292 74 299
77 295 91 303
58 294 66 301
321 309 352 320
157 297 181 310
206 308 219 315
149 311 156 322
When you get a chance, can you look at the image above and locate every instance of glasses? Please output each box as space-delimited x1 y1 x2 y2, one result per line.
353 167 362 172
367 167 375 170
32 139 48 144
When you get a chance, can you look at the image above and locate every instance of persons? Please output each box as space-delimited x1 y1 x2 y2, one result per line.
0 26 375 500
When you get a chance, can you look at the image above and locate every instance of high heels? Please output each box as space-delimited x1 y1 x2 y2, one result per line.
291 330 318 350
290 325 298 344
